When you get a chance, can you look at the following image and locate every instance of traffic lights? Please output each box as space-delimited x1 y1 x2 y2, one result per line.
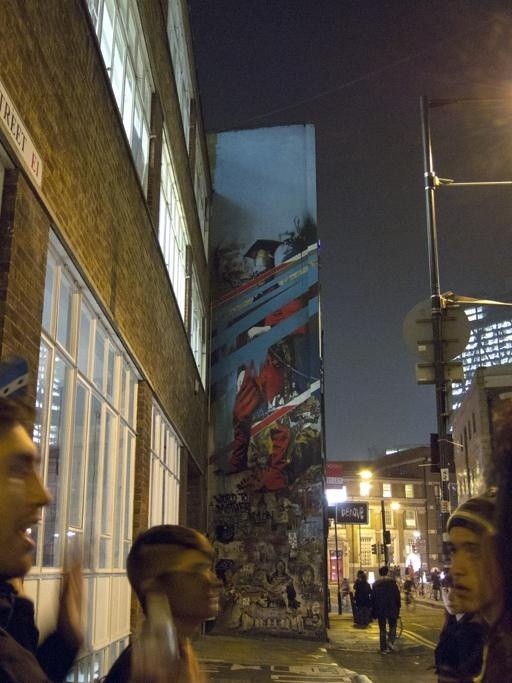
371 543 378 555
381 543 384 555
412 545 415 553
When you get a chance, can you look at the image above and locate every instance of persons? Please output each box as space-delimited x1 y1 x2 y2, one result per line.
373 564 402 653
335 560 443 616
0 393 89 683
210 343 325 636
430 570 489 683
100 523 226 681
445 493 512 681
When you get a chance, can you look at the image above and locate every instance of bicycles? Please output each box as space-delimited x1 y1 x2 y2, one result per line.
415 581 443 602
384 609 403 638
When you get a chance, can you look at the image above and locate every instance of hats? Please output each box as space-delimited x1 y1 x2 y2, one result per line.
446 484 502 537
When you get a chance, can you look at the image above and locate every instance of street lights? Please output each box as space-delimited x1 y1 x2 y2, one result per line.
358 455 431 478
